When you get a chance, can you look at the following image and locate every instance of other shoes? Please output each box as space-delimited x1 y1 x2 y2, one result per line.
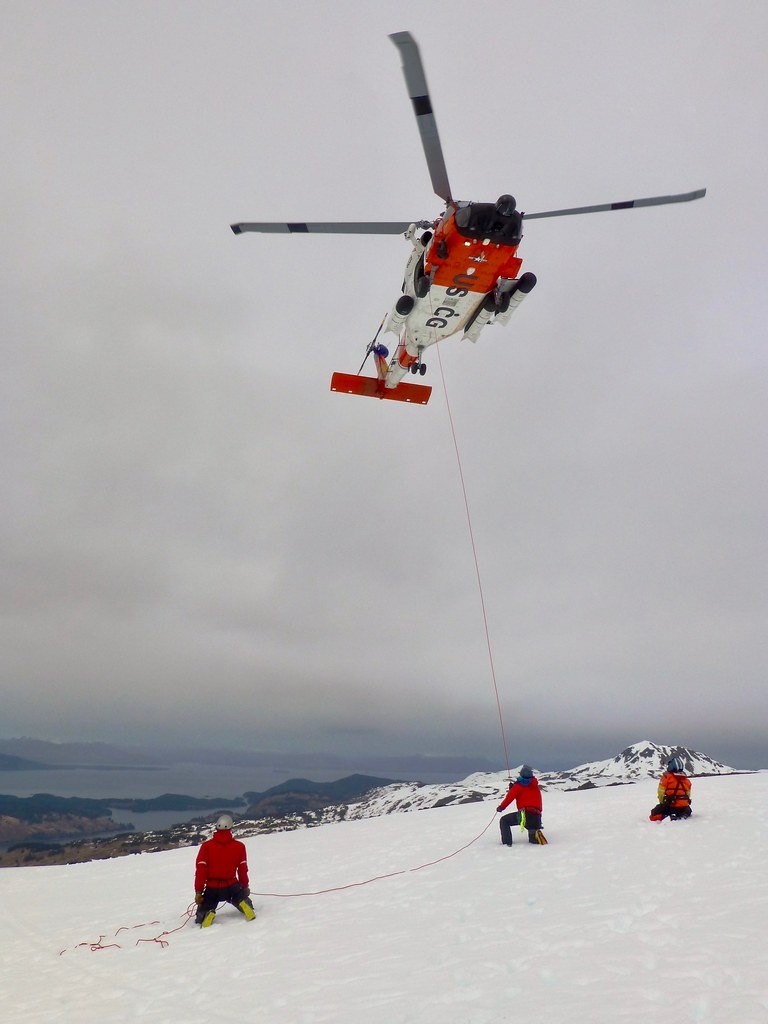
661 814 673 823
237 898 258 922
501 842 511 847
534 829 548 845
200 910 217 928
680 813 690 821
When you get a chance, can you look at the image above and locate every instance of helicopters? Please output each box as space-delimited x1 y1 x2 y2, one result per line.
229 32 709 406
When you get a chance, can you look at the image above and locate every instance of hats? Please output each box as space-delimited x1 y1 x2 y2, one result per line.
667 757 685 772
217 815 235 832
518 765 534 778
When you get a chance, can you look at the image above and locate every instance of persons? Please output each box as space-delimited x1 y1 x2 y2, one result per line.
650 757 692 821
194 815 256 928
498 765 548 847
